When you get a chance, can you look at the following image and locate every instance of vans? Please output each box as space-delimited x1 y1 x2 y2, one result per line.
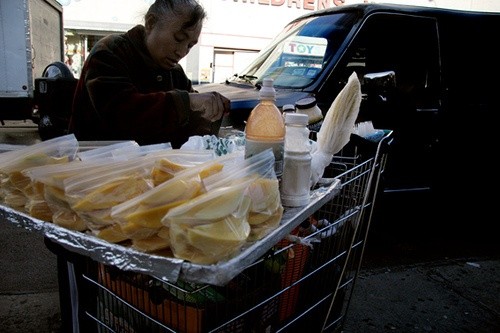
190 3 500 196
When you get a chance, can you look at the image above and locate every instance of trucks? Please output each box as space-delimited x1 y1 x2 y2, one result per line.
0 0 65 99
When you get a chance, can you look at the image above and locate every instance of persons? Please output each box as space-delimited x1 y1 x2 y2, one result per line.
44 0 232 333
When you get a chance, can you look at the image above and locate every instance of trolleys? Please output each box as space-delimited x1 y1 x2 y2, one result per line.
81 127 394 333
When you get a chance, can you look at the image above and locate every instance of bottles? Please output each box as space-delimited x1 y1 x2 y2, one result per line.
282 104 294 115
282 113 310 205
245 78 284 189
295 97 322 141
